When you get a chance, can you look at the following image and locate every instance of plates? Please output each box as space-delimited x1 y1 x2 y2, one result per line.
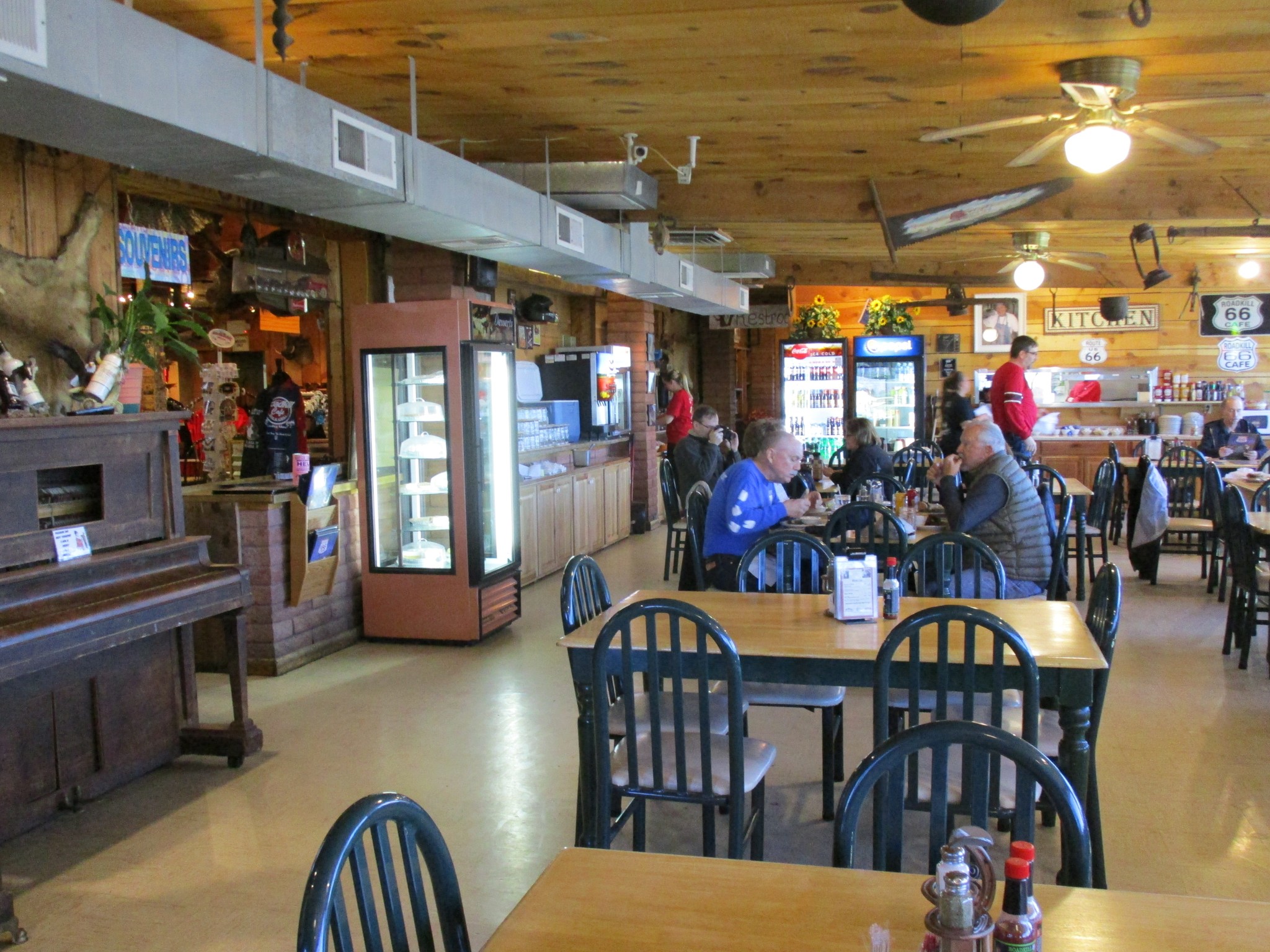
918 511 945 515
1158 413 1205 437
1242 476 1269 483
916 515 949 530
780 520 806 528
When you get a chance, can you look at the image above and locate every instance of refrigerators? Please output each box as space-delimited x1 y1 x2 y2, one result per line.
350 298 524 644
852 335 926 464
779 339 847 468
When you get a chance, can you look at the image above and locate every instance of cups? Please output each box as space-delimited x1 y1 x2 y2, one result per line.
835 479 919 541
292 453 309 485
573 450 591 468
517 407 568 451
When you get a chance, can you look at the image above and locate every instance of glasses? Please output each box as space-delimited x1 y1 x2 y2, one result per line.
696 421 713 429
1023 350 1037 356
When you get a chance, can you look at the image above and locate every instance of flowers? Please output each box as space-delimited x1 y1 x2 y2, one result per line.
789 294 920 339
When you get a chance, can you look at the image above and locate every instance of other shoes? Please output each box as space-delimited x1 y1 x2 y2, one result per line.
679 509 686 517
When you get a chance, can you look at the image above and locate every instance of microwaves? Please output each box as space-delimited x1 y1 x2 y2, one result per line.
1241 410 1270 436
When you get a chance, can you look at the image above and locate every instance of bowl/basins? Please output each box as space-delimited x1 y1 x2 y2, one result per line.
801 516 820 524
1052 429 1124 436
810 507 828 513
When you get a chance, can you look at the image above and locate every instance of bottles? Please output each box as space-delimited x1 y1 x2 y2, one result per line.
85 353 122 402
1154 370 1245 408
883 557 899 620
1126 417 1155 435
1167 438 1188 461
787 364 844 462
935 839 1043 952
0 340 46 411
857 361 916 451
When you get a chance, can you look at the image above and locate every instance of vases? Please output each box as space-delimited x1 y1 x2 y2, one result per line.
806 327 824 339
880 325 895 337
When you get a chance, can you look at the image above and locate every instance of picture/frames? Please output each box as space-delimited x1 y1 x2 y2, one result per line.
974 293 1026 353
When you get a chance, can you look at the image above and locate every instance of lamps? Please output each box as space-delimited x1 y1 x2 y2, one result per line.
1014 252 1045 290
1056 113 1141 173
281 336 305 360
239 211 257 244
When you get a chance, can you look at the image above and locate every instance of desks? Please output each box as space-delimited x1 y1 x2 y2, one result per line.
769 508 955 595
555 590 1110 889
1119 457 1260 544
476 848 1270 952
1038 477 1094 601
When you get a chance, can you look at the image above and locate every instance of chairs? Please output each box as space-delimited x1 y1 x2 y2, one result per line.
181 439 207 487
296 442 1270 952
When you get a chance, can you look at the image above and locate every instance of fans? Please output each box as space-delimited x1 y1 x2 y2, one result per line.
945 232 1107 273
918 55 1221 168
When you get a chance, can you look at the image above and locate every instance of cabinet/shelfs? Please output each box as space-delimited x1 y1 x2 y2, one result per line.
572 469 604 556
603 461 631 544
1156 401 1224 440
1036 441 1130 519
519 476 574 587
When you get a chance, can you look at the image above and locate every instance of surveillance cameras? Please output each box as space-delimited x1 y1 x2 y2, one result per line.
635 145 648 159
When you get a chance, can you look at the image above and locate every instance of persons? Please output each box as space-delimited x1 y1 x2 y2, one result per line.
1197 395 1269 518
655 335 1052 600
981 302 1018 345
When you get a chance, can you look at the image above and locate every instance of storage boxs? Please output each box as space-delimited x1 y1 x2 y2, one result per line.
515 360 581 442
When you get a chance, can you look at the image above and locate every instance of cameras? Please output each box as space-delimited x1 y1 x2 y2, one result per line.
715 425 736 441
